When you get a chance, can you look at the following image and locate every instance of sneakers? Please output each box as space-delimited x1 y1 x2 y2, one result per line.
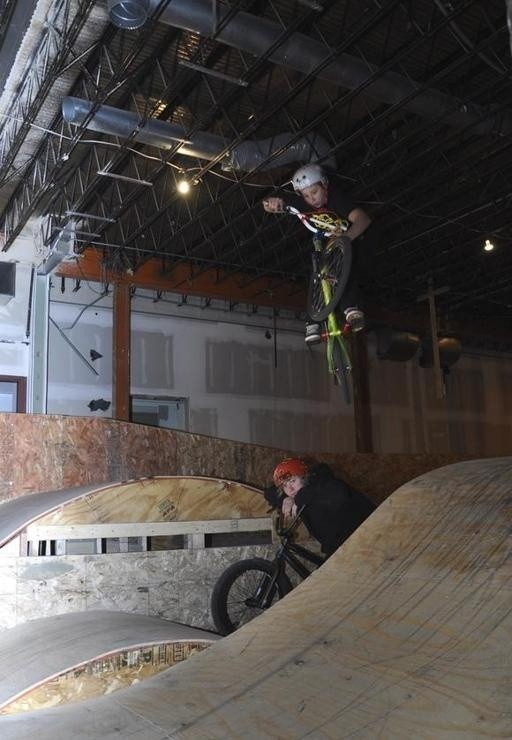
344 306 365 332
305 323 321 342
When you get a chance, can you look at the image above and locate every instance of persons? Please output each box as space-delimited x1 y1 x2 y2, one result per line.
264 458 377 561
262 160 372 344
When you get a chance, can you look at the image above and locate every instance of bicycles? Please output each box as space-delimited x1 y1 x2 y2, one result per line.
262 199 369 406
211 500 326 638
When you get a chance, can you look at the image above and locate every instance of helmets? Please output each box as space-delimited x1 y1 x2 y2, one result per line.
292 163 329 196
273 458 308 487
